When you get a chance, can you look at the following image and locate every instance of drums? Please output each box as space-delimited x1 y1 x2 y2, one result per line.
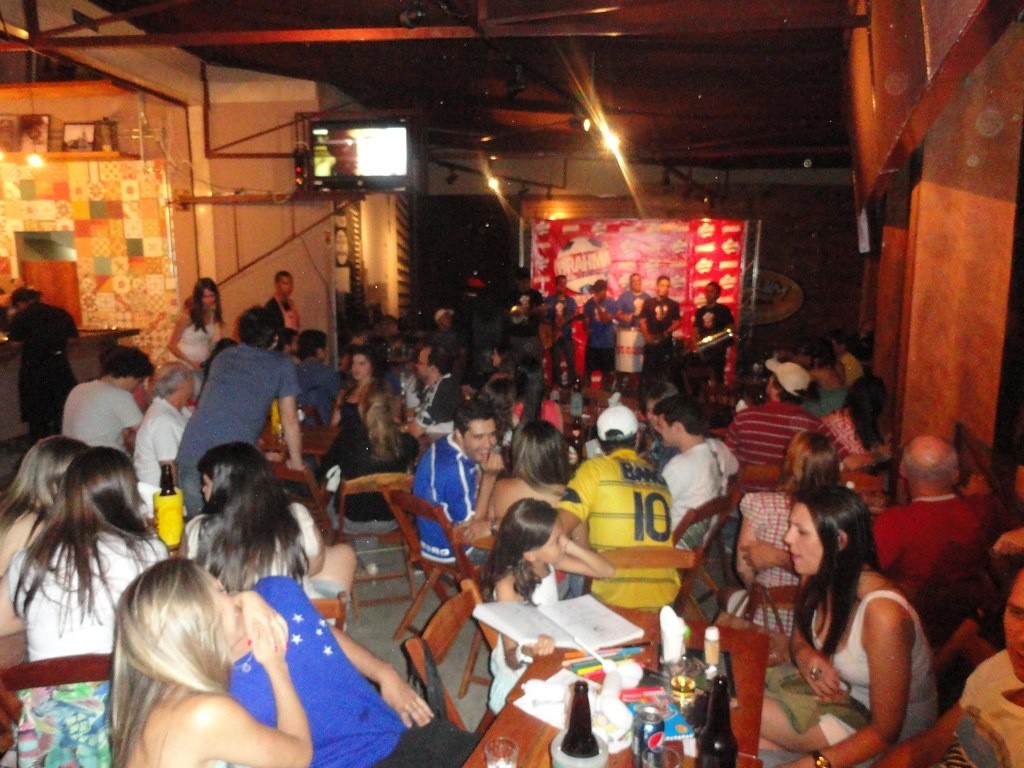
615 328 644 371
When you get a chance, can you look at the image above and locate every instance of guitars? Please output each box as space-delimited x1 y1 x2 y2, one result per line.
508 293 566 324
538 311 590 348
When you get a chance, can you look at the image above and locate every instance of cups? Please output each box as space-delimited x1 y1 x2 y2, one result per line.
641 745 681 768
665 655 706 725
484 735 519 768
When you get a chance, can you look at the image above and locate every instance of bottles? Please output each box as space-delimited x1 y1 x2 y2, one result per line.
569 376 582 417
549 384 560 403
568 418 583 465
695 673 738 768
561 680 599 758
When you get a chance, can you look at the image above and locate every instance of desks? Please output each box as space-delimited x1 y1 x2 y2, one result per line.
462 601 773 768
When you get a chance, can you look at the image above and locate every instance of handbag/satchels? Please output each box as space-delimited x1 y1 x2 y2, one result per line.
764 661 873 733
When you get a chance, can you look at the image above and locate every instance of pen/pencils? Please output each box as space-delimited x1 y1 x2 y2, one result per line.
562 640 651 675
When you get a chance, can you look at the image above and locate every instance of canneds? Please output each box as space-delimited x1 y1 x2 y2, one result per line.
633 707 665 768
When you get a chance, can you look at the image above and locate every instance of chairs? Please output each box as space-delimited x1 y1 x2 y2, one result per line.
0 369 994 739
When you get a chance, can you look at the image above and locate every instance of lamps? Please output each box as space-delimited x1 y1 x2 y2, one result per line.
662 169 715 209
545 188 554 200
570 115 591 132
398 8 429 28
500 81 525 99
445 169 458 185
518 182 530 199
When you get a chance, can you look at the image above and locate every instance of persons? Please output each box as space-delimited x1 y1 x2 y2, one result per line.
1 271 1024 715
758 486 939 768
0 445 167 768
867 561 1024 767
111 558 313 768
230 575 484 768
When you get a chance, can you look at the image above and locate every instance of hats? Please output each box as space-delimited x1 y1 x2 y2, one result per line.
763 358 810 399
595 405 639 441
434 308 455 322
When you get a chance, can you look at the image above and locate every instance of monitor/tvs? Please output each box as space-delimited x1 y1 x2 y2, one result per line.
311 116 420 191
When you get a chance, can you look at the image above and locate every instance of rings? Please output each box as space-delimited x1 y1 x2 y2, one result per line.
811 667 819 675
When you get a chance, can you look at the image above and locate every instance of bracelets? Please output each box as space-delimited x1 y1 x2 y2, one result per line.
812 750 831 768
490 517 496 535
